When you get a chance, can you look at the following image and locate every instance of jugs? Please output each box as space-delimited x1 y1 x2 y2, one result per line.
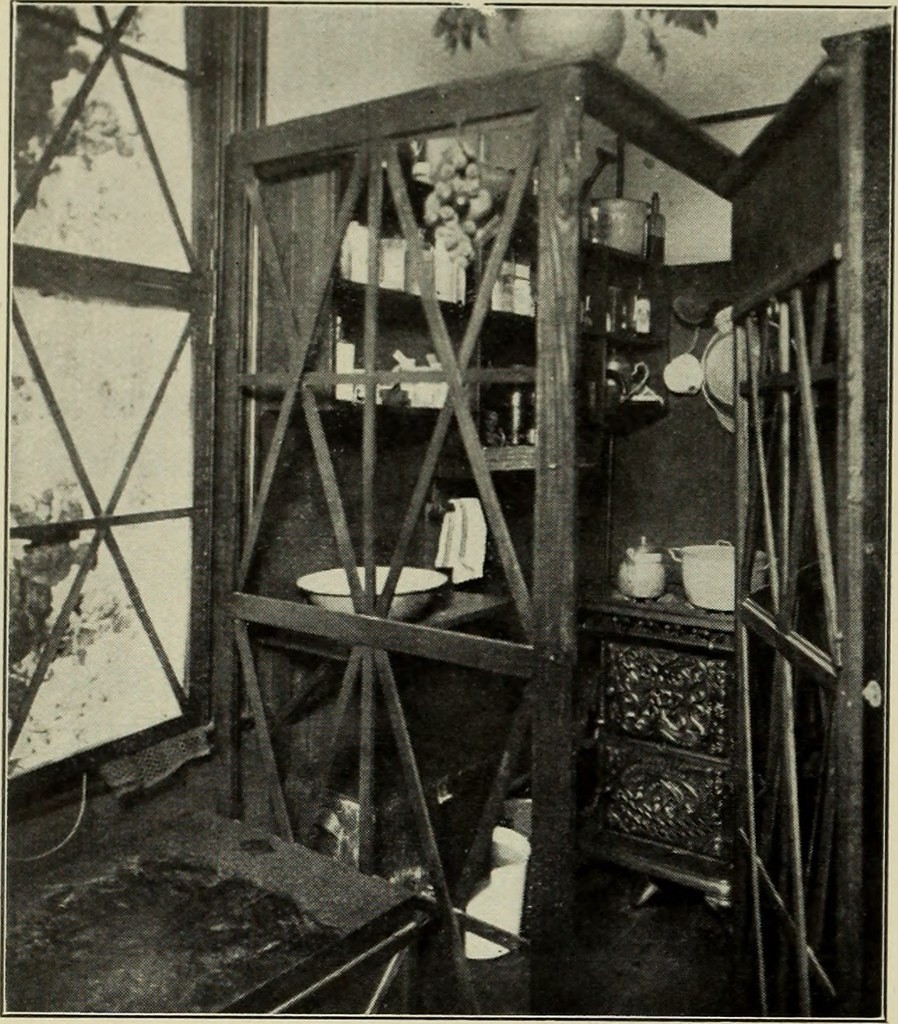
618 535 666 599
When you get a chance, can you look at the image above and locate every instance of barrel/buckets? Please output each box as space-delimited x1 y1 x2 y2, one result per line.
464 826 530 958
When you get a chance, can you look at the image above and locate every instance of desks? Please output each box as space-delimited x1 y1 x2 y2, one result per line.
6 811 435 1015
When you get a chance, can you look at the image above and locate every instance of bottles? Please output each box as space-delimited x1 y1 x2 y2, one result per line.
644 192 666 263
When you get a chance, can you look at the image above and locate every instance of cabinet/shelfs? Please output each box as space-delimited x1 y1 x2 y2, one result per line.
259 158 670 432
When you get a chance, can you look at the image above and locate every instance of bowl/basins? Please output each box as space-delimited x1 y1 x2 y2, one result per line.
296 563 449 621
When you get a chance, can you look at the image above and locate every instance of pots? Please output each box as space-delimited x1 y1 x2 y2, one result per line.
669 538 766 612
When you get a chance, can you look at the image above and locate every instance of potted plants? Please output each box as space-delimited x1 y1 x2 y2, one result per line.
432 5 719 77
